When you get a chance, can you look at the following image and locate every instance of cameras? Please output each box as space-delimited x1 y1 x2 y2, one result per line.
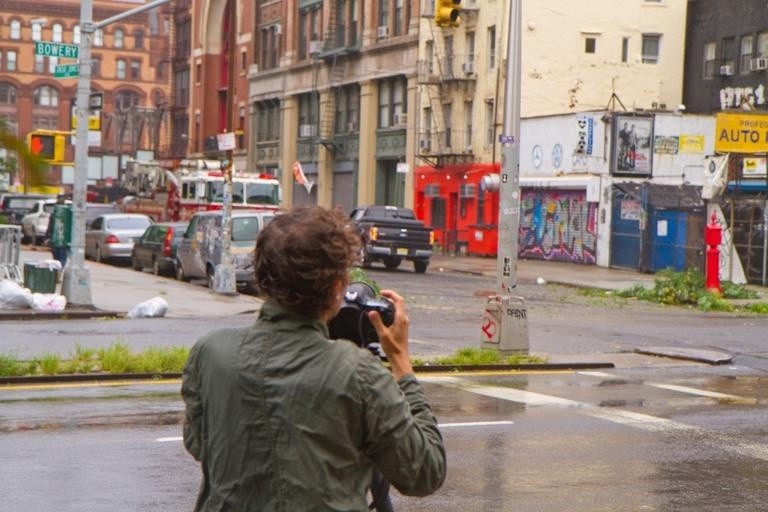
327 280 396 362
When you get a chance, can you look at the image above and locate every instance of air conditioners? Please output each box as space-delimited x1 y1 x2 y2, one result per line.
273 23 283 35
750 57 767 72
393 113 407 127
299 124 314 137
719 65 734 76
376 26 389 39
461 62 474 76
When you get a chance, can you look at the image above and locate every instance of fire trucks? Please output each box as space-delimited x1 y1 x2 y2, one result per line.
118 160 283 250
56 178 129 208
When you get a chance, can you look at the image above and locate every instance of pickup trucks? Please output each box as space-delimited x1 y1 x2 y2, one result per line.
342 206 434 275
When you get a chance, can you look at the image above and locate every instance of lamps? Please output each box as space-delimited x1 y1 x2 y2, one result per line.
601 92 627 123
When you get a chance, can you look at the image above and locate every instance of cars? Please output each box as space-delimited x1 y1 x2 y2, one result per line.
84 211 155 266
128 221 196 278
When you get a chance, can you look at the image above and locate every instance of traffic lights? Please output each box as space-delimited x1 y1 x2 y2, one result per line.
435 0 462 28
25 128 76 166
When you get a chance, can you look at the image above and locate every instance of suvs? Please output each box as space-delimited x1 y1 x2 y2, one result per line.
0 193 47 226
21 199 74 243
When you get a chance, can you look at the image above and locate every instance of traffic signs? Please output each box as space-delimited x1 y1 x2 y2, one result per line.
54 63 79 78
34 41 78 58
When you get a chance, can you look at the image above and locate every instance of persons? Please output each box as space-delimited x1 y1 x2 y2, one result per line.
617 120 631 173
179 204 447 512
44 195 72 286
628 123 640 171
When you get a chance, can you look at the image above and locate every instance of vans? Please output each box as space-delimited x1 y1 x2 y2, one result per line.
173 208 289 285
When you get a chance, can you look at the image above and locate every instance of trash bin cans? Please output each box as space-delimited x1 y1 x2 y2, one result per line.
23 260 61 293
